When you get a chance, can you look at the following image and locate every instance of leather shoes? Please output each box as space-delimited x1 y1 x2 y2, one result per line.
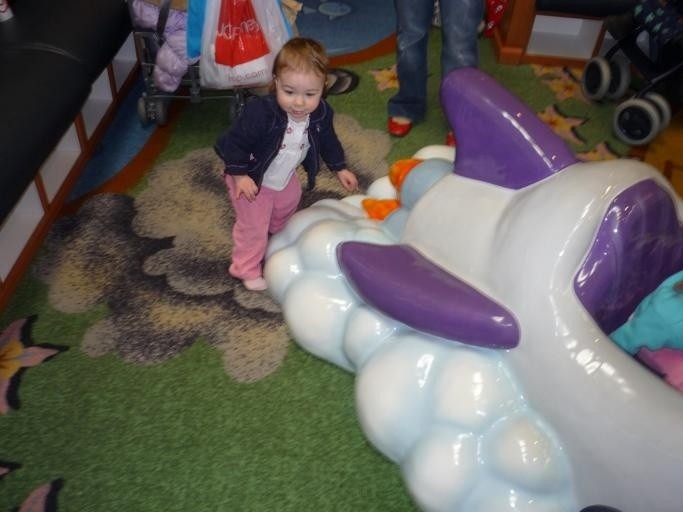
388 115 414 137
445 129 457 149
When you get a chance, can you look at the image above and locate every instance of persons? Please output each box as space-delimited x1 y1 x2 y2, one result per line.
387 0 484 147
212 38 360 291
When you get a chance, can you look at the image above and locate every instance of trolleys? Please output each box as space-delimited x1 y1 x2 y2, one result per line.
135 1 260 129
